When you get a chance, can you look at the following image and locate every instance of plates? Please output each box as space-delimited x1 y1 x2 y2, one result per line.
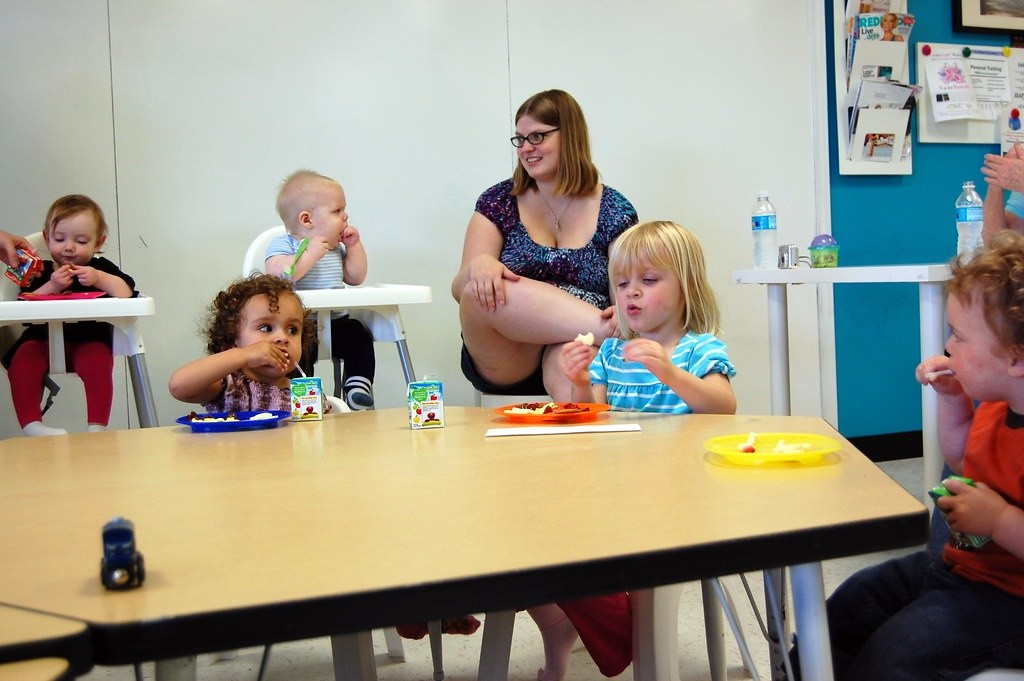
17 291 106 300
703 432 842 467
175 410 290 434
493 401 612 425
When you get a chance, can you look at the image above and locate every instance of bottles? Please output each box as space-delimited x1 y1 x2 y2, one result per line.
955 181 984 257
751 192 778 270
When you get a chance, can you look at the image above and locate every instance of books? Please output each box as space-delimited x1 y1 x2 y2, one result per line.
843 0 919 163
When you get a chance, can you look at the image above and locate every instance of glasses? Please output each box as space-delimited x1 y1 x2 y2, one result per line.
510 127 560 148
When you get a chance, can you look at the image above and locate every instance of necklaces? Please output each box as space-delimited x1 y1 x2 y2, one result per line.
536 186 572 230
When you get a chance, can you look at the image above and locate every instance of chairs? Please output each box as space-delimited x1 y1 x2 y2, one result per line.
0 230 159 428
245 225 442 681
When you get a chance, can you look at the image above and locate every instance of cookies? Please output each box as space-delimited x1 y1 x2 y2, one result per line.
555 409 580 414
504 402 559 414
574 332 594 346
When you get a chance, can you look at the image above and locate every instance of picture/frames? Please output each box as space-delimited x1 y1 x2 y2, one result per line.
950 1 1024 39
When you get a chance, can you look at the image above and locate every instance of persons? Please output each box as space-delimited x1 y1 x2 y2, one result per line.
980 140 1024 242
263 170 375 410
167 274 480 641
451 89 639 405
522 221 736 681
881 16 902 43
781 228 1024 681
0 194 140 438
0 231 44 278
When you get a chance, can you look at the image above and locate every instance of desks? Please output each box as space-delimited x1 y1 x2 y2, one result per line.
733 264 954 681
1 415 933 681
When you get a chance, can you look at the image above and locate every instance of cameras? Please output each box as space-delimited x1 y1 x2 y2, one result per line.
777 245 799 269
